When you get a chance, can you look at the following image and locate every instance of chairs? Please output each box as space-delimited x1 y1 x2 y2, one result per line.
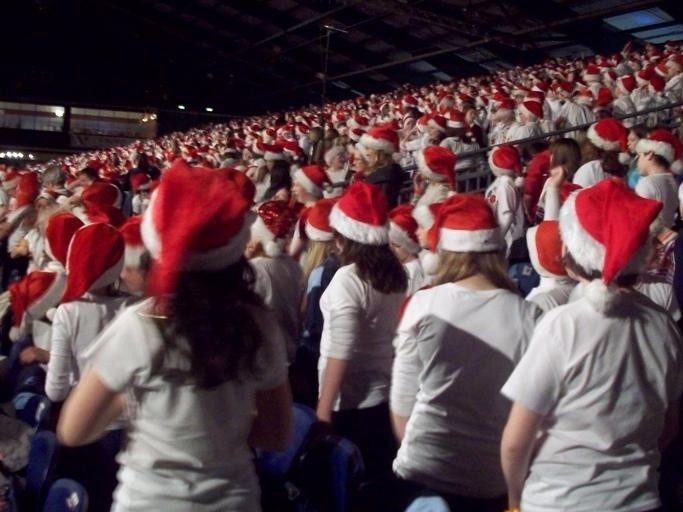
510 263 539 292
0 340 449 512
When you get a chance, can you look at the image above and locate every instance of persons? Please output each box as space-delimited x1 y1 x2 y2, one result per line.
2 39 683 512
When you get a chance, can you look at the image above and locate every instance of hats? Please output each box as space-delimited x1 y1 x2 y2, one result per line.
524 40 682 118
259 79 523 170
386 204 423 254
259 200 298 257
141 158 255 322
422 193 503 275
524 119 681 317
488 144 523 187
4 168 147 342
418 146 458 196
305 198 339 242
328 180 390 245
294 164 334 198
412 202 442 231
